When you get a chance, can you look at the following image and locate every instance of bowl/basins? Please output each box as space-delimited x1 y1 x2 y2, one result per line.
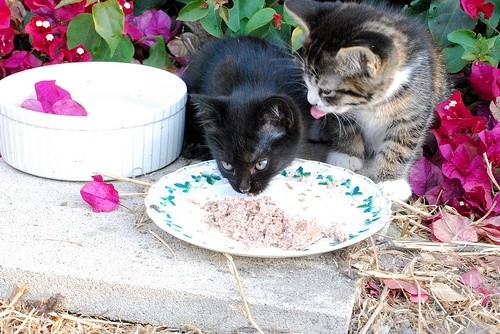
0 60 187 182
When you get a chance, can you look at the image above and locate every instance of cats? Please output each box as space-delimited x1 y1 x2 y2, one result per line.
181 32 325 195
297 0 442 201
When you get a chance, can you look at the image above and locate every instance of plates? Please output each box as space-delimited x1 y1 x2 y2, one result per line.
144 157 392 258
